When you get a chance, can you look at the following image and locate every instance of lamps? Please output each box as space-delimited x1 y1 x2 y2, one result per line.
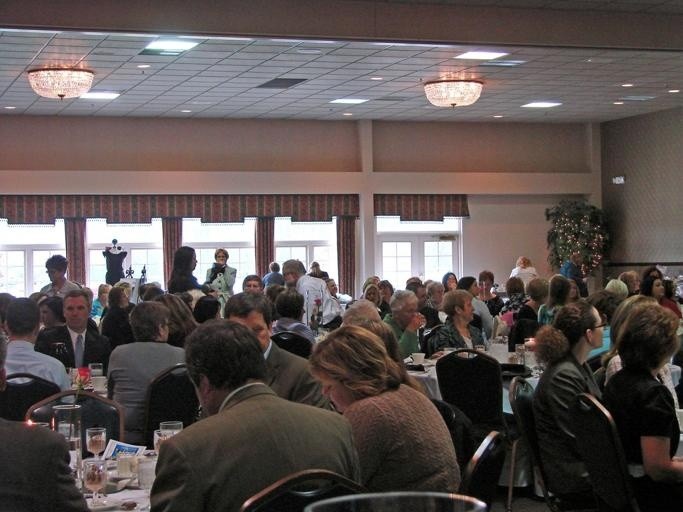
424 79 484 107
27 69 95 98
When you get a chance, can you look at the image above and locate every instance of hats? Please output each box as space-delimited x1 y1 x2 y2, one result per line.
309 261 319 269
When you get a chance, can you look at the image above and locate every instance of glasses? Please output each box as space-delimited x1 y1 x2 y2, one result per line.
585 321 608 331
45 269 59 275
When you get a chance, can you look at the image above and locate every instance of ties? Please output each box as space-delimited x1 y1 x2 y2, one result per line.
73 334 85 368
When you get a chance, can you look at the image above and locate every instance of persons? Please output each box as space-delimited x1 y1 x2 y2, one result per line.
585 278 628 325
257 262 285 292
558 251 589 298
114 282 136 316
137 280 164 304
425 282 450 325
5 296 75 414
442 272 458 291
357 319 423 397
431 289 491 358
306 324 462 511
537 275 571 326
376 278 395 306
282 260 344 332
195 294 221 324
404 283 440 328
639 275 665 302
0 325 94 512
507 256 540 290
382 288 428 362
171 291 193 308
323 277 344 309
150 317 363 512
364 285 391 320
39 255 80 302
222 292 331 415
29 292 43 305
88 283 109 323
602 293 679 411
37 294 63 332
267 293 315 357
510 279 550 349
262 285 287 300
35 288 110 371
603 302 682 512
305 261 328 278
454 276 494 339
343 301 380 330
151 293 201 348
527 297 603 511
566 279 581 305
99 287 132 347
618 271 636 298
641 266 663 279
110 300 190 450
241 274 266 296
204 247 236 319
188 288 205 307
167 245 207 297
475 270 504 316
661 277 683 350
0 292 11 324
361 275 380 296
408 277 421 285
495 275 529 334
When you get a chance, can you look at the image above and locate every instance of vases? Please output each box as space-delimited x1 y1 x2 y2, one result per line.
52 405 83 490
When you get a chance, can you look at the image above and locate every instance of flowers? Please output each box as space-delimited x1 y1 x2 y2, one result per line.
71 367 91 406
314 299 324 337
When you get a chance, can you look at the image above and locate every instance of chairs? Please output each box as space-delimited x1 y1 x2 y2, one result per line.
508 375 558 512
436 349 503 424
239 469 369 512
145 363 200 449
461 430 507 512
568 393 641 512
27 389 125 458
1 373 61 422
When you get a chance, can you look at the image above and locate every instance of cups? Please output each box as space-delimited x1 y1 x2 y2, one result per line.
137 457 156 491
475 344 485 353
116 452 138 480
411 352 425 365
86 427 107 459
443 347 456 357
82 459 108 508
515 344 526 365
155 429 175 459
88 363 103 378
160 422 184 433
411 313 426 329
